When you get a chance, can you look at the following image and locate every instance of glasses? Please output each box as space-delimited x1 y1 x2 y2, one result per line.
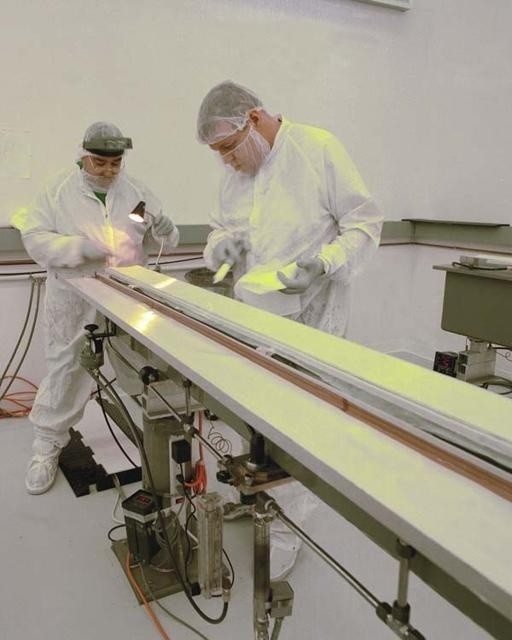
85 155 127 173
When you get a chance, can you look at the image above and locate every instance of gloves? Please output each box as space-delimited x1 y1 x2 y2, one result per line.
152 214 172 236
212 238 241 269
277 260 323 295
84 238 110 261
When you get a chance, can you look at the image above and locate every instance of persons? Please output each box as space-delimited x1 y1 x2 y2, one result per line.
195 78 382 586
18 121 181 494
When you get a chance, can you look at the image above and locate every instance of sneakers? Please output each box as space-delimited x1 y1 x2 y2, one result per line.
27 442 59 494
220 492 257 520
269 531 302 582
